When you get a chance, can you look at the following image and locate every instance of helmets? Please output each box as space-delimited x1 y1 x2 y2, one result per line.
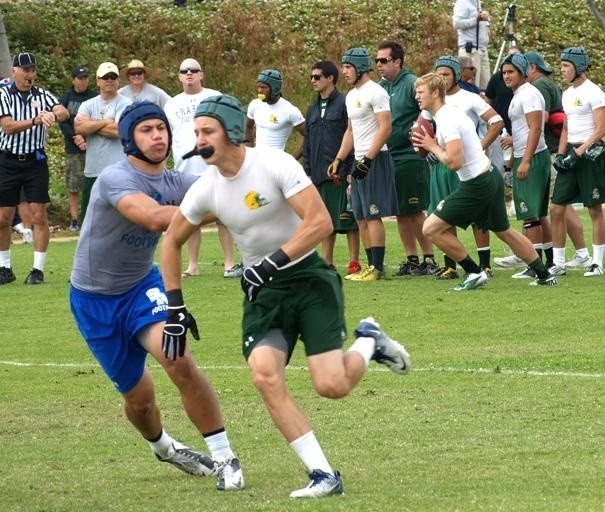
194 92 248 146
256 68 282 102
116 98 173 163
341 48 373 84
500 46 590 83
434 54 463 85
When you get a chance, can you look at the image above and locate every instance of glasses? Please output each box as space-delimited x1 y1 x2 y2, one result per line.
180 67 202 73
309 74 323 80
130 71 144 76
375 57 393 65
98 73 118 80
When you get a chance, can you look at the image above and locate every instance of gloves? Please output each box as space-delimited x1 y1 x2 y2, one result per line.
563 148 579 168
239 249 291 301
159 290 199 362
326 156 344 181
554 153 567 172
352 157 371 181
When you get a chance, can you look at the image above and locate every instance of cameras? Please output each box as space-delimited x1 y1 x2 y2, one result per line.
505 2 518 22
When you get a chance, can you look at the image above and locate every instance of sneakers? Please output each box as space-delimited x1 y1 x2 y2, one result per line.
222 263 243 278
23 267 44 285
69 220 79 231
152 438 218 478
0 266 16 285
345 254 605 291
212 456 246 491
355 313 411 377
288 468 344 501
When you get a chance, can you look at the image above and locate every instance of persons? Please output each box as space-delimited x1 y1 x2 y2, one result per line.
0 53 69 285
377 41 605 291
164 56 237 277
224 70 309 277
54 67 98 232
302 61 361 276
326 46 401 282
452 1 491 88
116 59 172 108
74 62 133 176
69 100 244 489
160 94 411 499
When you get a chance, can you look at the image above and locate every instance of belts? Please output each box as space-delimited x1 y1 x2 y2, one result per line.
16 154 30 161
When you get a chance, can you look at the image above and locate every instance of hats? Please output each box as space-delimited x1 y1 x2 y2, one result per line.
70 66 90 77
95 61 120 78
120 58 151 79
12 52 39 71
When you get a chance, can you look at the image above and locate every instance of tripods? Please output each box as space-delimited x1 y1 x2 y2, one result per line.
491 35 517 75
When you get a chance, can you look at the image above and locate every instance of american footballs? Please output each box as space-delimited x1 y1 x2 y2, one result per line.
411 115 433 158
